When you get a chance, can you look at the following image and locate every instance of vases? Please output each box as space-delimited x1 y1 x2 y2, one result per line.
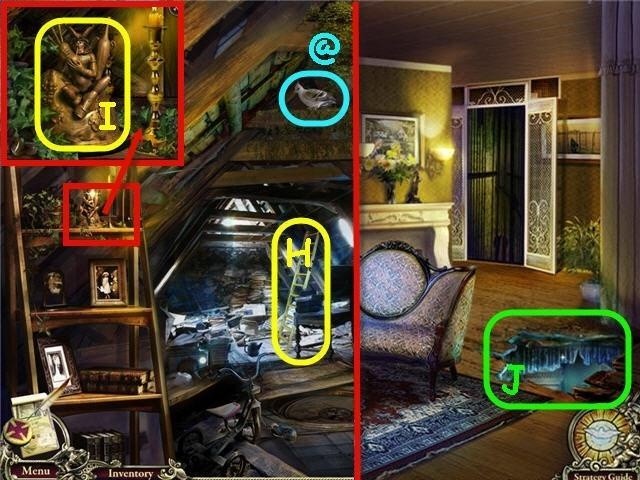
382 175 410 204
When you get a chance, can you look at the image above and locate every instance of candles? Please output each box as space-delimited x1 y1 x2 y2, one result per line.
148 5 163 27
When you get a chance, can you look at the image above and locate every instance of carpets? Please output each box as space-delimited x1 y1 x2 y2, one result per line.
361 353 554 480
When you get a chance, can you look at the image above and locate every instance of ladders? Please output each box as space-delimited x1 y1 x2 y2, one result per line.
278 230 320 355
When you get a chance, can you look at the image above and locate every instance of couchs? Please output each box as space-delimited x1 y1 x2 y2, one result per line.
359 240 477 402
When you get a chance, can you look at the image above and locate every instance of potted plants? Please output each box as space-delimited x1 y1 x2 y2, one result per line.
554 216 601 306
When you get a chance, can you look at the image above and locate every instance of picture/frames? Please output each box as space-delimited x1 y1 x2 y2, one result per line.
359 112 425 174
556 112 601 165
37 335 82 398
88 258 129 308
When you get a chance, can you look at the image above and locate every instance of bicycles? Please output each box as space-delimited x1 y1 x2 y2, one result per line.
175 352 298 477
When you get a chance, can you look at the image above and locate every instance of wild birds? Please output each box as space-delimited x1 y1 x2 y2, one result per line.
286 82 337 116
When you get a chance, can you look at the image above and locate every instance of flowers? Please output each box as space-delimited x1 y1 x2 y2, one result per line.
359 140 420 179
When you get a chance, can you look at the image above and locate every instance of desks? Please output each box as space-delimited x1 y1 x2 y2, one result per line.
294 309 351 359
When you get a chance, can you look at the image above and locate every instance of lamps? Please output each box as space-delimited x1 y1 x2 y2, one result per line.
426 145 455 182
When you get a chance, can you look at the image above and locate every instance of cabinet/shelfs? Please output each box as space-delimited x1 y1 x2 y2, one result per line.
6 165 176 467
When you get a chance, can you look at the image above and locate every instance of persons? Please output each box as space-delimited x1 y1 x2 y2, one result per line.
58 35 113 121
50 353 64 378
101 271 112 298
